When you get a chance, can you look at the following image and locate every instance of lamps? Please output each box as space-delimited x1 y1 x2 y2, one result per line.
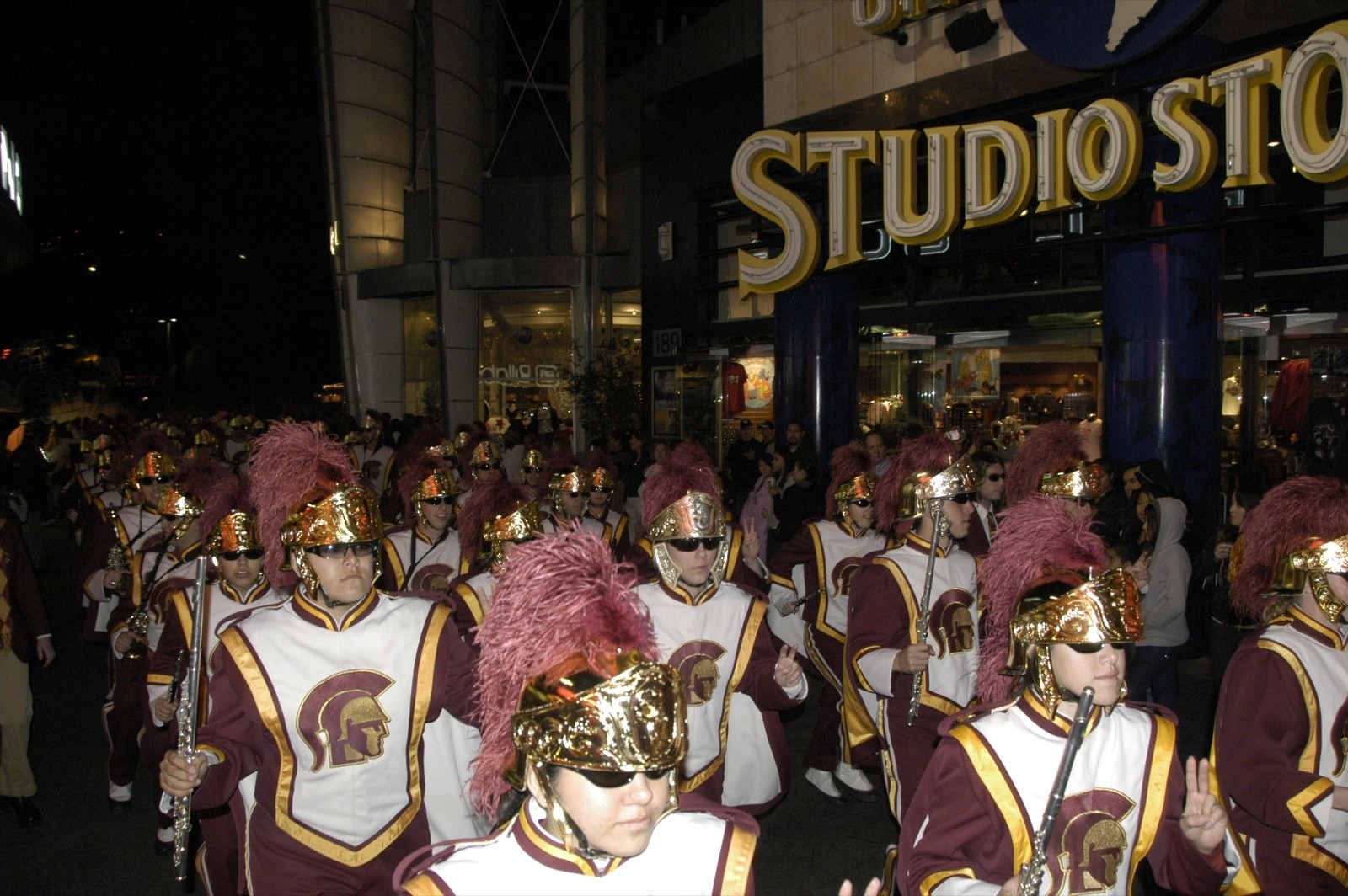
944 9 1000 52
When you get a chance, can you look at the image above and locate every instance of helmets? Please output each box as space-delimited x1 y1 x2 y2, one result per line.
1229 476 1348 622
1002 419 1103 506
974 492 1144 721
82 416 618 601
873 429 978 537
462 512 691 860
826 443 877 521
640 441 730 590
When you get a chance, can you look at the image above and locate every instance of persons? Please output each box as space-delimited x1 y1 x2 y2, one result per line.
618 418 1348 896
1201 480 1348 896
1076 412 1103 466
0 359 629 896
392 525 880 895
894 494 1240 894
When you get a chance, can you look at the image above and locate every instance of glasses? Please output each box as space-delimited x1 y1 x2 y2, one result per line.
848 499 874 507
589 485 610 493
561 491 589 498
1066 643 1134 654
476 462 501 470
138 476 173 485
987 473 1006 481
655 537 722 552
940 491 982 504
554 765 678 789
1064 497 1102 506
161 513 185 522
503 536 536 545
304 540 377 559
525 466 543 474
219 549 264 561
421 496 454 506
